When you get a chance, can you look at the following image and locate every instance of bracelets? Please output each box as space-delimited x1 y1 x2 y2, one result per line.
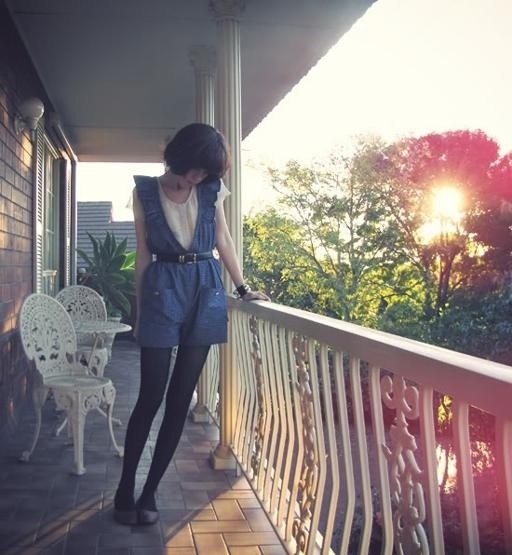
237 283 251 297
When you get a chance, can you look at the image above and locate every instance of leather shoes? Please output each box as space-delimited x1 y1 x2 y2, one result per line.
137 496 160 525
114 492 139 526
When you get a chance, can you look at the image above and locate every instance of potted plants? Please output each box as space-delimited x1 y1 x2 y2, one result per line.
73 230 138 346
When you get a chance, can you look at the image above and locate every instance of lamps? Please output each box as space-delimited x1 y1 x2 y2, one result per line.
15 95 45 134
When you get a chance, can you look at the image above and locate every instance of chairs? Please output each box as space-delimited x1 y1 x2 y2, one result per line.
19 292 125 474
56 284 109 378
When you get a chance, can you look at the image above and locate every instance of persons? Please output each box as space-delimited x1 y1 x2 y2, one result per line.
112 121 272 525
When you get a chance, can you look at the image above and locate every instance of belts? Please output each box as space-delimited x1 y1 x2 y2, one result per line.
158 251 215 265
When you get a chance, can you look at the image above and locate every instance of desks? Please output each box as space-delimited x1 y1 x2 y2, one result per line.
53 321 133 437
